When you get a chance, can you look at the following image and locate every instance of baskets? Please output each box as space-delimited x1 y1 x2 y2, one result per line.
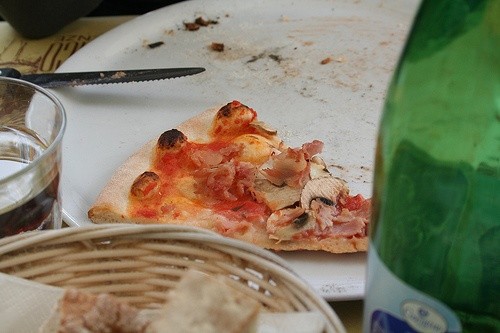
0 221 349 332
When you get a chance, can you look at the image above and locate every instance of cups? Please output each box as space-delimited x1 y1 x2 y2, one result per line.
0 77 66 238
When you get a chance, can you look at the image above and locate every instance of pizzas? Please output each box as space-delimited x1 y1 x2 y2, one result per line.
86 98 371 253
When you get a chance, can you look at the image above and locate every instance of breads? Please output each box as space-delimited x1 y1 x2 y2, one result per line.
51 267 264 333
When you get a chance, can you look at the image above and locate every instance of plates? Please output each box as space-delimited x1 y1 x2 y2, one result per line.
27 0 422 301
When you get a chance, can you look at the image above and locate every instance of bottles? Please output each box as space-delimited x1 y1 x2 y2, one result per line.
363 0 500 333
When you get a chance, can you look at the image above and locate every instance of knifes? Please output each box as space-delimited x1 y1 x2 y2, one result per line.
0 67 206 88
